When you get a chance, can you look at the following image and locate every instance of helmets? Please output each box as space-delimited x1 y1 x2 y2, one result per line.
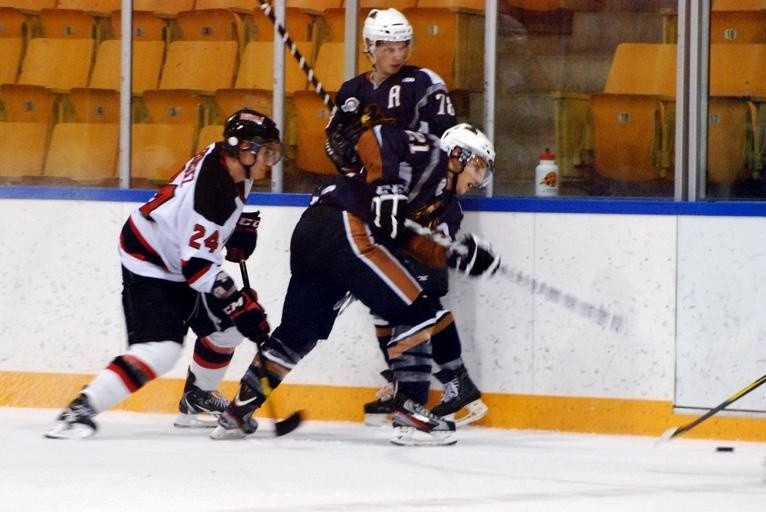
438 122 497 169
362 7 413 46
222 108 281 155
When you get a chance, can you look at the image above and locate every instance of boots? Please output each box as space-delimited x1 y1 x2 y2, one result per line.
362 368 401 415
428 363 482 419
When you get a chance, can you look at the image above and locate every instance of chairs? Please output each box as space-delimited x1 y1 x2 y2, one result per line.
553 1 765 192
1 1 499 194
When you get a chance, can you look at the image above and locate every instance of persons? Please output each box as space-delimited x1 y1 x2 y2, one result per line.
323 8 483 417
217 120 498 434
54 107 282 426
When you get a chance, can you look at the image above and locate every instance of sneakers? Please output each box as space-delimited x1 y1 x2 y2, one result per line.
217 382 266 435
178 364 234 417
56 394 97 432
391 393 456 436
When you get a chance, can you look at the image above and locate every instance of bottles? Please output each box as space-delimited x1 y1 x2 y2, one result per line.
534 145 560 198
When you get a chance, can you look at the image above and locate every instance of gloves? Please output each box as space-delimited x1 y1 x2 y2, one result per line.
220 287 271 342
222 207 264 265
367 176 410 240
446 232 500 281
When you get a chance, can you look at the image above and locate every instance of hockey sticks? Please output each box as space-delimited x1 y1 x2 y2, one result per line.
660 375 766 441
238 248 302 437
